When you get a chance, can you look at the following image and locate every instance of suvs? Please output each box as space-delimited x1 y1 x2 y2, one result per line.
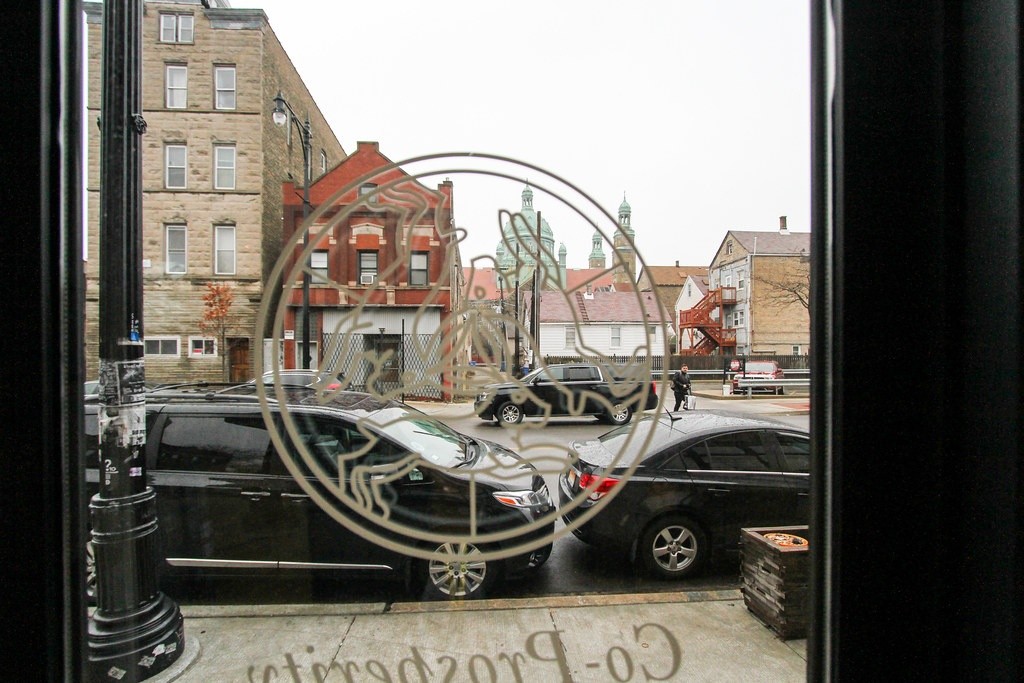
247 369 355 392
473 363 659 424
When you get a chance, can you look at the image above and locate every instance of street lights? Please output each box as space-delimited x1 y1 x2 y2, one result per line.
273 90 311 370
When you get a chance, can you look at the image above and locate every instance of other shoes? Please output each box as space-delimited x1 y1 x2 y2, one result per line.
684 405 688 409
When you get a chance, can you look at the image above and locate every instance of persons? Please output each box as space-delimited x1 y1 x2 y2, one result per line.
673 363 691 411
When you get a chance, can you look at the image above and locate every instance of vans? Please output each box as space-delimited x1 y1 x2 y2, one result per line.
83 382 557 606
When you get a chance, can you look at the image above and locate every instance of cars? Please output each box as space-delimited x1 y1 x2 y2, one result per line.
82 380 152 400
733 361 784 394
559 408 810 578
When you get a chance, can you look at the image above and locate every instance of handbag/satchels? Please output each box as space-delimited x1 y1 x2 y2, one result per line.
670 371 682 391
